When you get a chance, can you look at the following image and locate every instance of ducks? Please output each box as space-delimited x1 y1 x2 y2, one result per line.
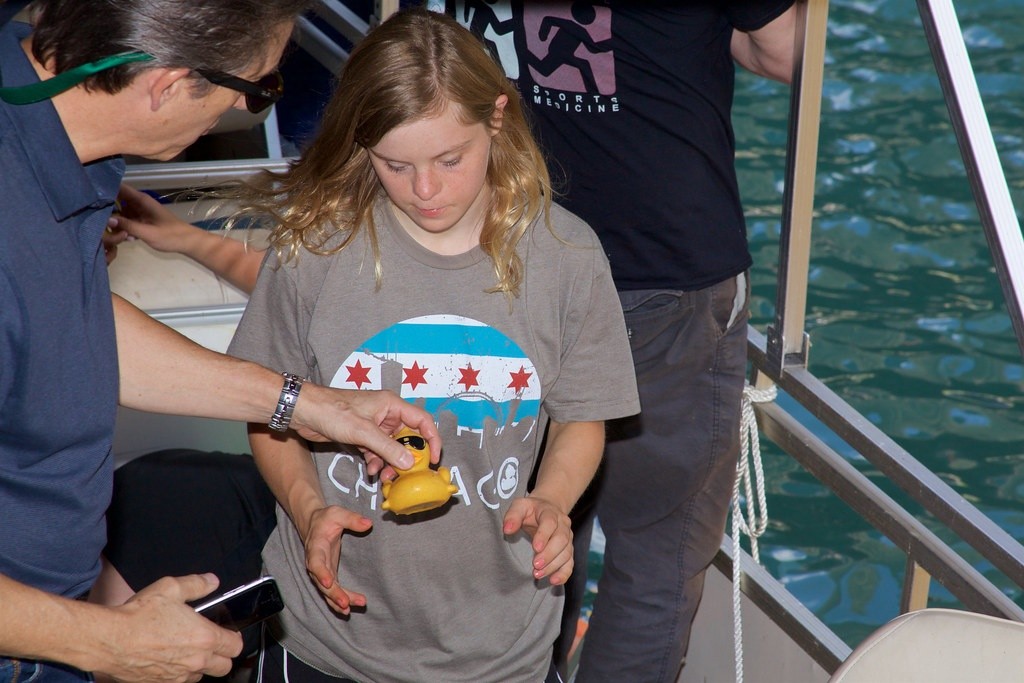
381 426 458 516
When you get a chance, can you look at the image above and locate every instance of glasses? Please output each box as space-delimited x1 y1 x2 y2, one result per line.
178 62 285 114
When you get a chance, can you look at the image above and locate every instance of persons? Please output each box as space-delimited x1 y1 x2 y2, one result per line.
0 1 443 683
398 0 810 683
223 7 642 683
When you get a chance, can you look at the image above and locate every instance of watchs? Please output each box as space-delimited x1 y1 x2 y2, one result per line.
267 372 306 432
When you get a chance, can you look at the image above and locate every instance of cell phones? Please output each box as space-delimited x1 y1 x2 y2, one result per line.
193 576 285 626
